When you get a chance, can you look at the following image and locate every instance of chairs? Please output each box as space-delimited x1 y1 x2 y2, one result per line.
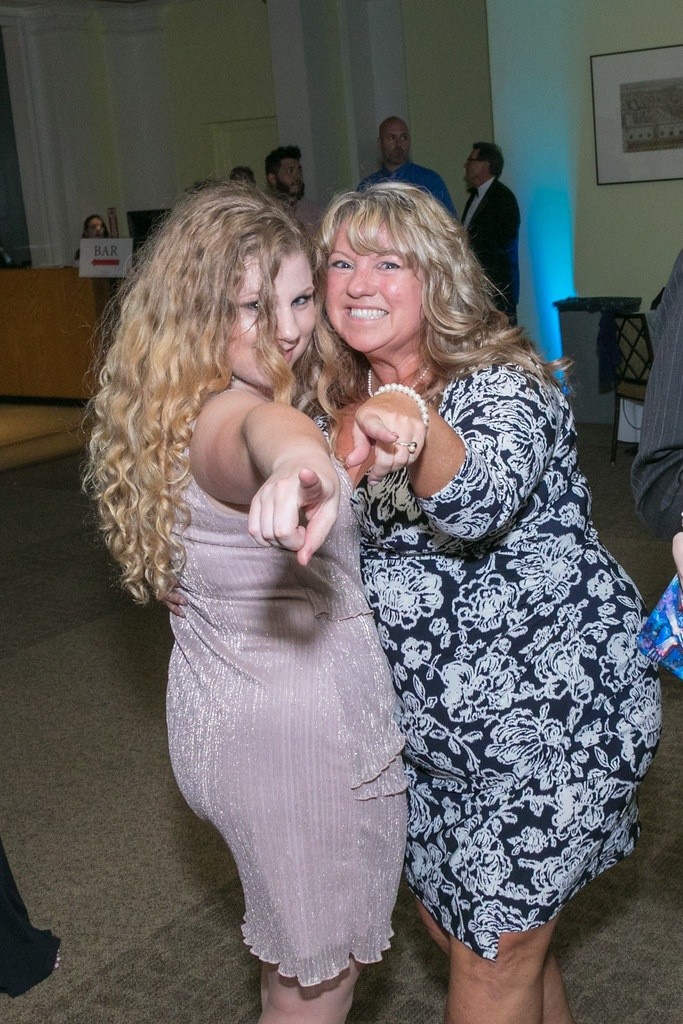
606 312 656 467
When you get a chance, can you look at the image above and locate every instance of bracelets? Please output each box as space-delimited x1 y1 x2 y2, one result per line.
373 382 429 428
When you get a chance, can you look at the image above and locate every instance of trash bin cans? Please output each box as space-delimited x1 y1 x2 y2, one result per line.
557 296 642 424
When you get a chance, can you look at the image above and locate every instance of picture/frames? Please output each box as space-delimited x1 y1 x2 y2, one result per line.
589 43 683 186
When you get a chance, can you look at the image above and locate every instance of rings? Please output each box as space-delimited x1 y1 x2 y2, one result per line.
396 441 417 454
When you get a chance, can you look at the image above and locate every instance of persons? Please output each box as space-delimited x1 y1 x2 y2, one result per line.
72 214 110 267
630 248 683 592
294 181 663 1024
356 116 459 221
77 179 411 1023
229 166 256 186
460 141 521 327
262 145 304 213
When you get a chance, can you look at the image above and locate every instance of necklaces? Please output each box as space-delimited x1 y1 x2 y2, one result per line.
367 361 432 397
